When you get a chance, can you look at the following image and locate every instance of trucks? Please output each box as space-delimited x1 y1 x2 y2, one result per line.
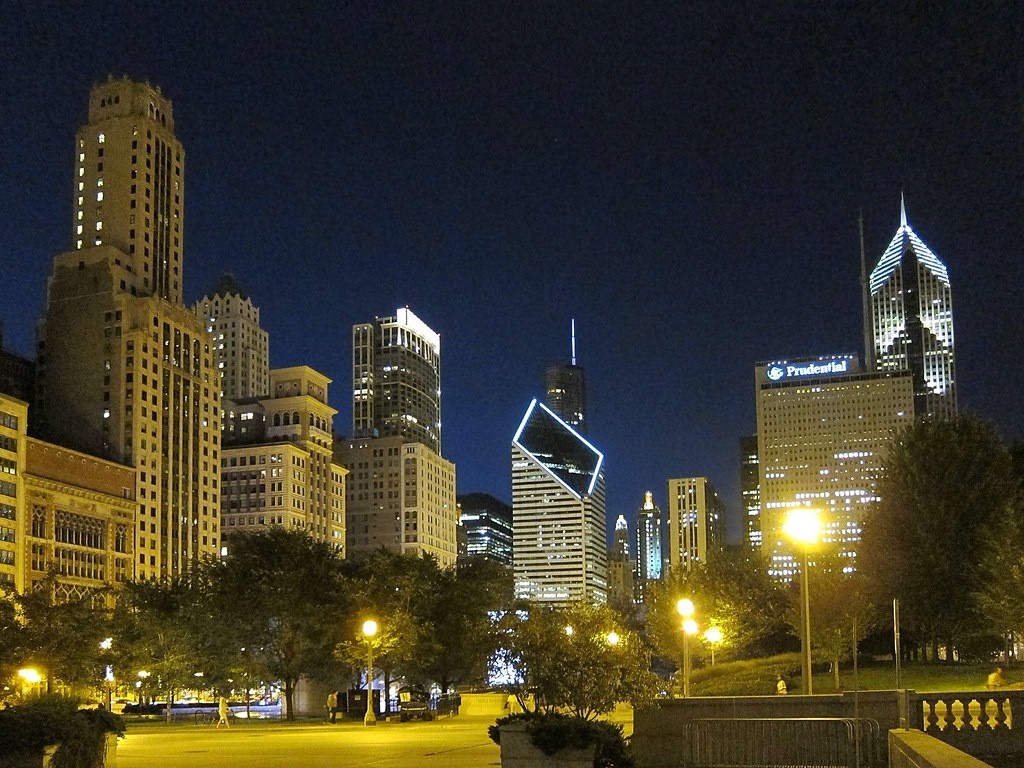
398 682 461 722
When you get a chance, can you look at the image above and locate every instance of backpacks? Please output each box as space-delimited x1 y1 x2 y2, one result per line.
327 694 333 707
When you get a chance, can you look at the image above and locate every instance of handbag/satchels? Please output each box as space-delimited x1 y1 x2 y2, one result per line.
330 712 333 718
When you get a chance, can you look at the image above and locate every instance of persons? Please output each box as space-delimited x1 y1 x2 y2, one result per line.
775 675 787 695
988 668 1005 691
84 700 105 710
507 692 518 714
323 691 338 724
216 694 230 729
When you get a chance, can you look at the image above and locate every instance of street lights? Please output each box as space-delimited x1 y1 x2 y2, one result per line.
677 598 699 699
706 628 718 667
361 620 377 725
787 511 820 695
